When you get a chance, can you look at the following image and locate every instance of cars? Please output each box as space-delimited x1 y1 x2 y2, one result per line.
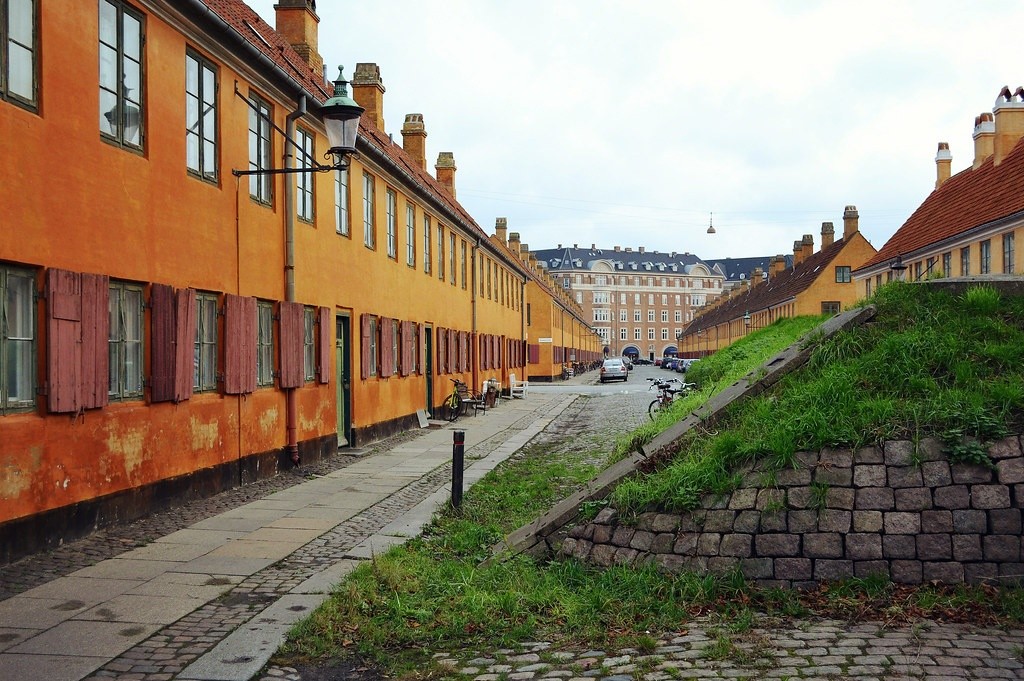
608 357 634 370
654 358 688 373
600 359 628 383
685 360 700 370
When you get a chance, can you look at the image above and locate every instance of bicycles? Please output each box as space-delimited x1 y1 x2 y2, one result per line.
441 379 468 422
646 377 696 422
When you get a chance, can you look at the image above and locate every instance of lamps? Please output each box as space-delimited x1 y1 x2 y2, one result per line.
104 72 139 141
744 310 766 329
232 63 366 178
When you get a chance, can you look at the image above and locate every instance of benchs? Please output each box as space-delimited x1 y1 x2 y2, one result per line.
509 373 529 398
454 383 487 417
